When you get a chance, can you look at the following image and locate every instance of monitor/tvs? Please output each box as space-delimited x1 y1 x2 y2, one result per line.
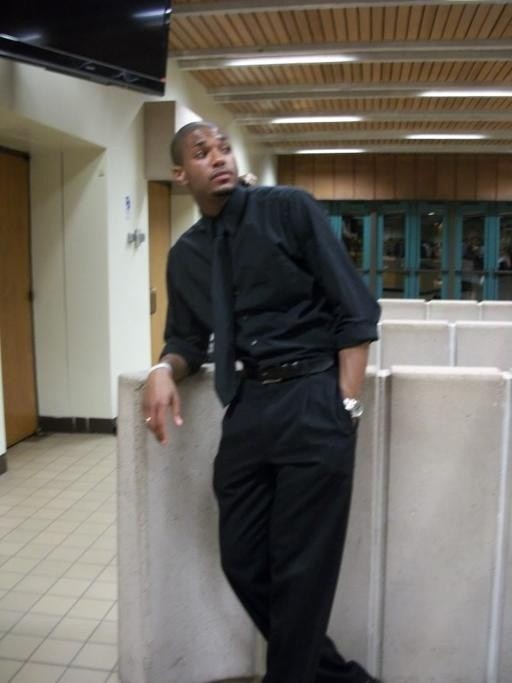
0 0 173 95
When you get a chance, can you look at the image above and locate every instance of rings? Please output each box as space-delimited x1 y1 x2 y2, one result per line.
143 415 152 423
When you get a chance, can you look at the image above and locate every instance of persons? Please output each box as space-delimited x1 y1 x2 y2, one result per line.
139 118 385 680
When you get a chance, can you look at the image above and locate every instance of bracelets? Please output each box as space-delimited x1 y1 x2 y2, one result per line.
147 362 173 374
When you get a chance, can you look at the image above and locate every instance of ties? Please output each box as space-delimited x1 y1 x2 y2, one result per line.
204 220 241 406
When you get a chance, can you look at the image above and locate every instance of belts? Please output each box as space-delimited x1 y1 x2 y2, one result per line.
244 351 338 383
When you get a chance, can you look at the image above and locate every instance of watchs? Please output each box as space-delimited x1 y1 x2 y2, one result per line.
341 395 365 419
